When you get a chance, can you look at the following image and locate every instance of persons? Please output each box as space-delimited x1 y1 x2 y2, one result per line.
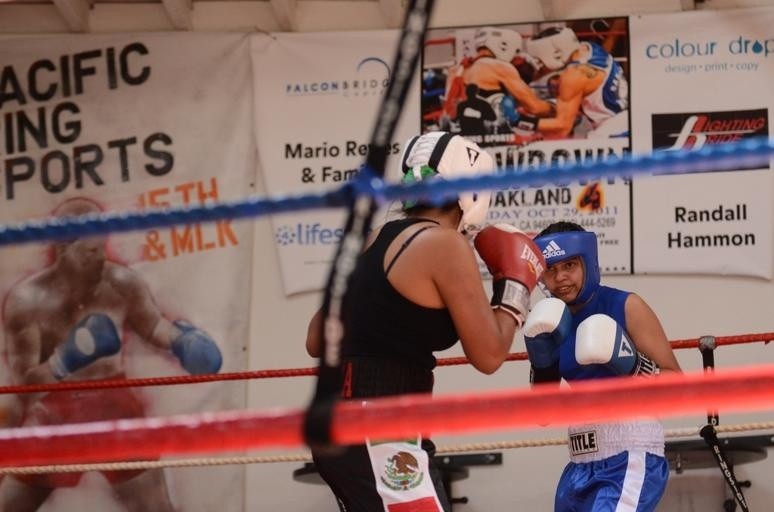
443 24 556 132
1 194 225 512
496 24 629 142
520 220 686 512
293 128 549 512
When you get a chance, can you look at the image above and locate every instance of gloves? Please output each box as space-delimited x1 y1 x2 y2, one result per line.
44 313 119 382
573 311 659 384
473 221 545 325
170 317 222 377
521 298 574 371
492 95 540 132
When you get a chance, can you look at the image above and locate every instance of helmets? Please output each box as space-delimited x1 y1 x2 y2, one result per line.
525 26 579 70
398 131 494 226
530 230 598 303
473 27 522 62
